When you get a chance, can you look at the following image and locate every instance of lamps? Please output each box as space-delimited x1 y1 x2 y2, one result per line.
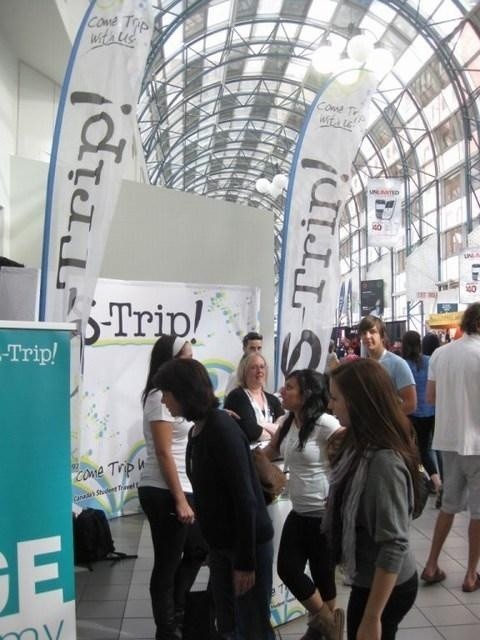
255 163 289 198
313 25 394 87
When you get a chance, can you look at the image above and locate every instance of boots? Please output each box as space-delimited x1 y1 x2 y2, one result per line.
149 586 186 640
301 603 344 639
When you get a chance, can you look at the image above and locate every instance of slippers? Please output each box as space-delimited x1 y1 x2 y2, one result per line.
421 566 445 583
462 572 480 593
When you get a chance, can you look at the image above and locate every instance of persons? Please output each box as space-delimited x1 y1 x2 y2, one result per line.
134 300 480 639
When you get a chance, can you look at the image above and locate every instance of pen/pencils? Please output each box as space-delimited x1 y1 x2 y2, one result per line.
170 513 197 521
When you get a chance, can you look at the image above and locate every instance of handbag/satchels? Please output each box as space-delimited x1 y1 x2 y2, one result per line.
250 446 287 506
412 470 431 520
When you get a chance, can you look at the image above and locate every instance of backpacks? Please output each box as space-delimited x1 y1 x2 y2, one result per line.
73 507 127 568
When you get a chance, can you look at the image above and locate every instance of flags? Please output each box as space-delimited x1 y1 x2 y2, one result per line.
367 175 404 248
